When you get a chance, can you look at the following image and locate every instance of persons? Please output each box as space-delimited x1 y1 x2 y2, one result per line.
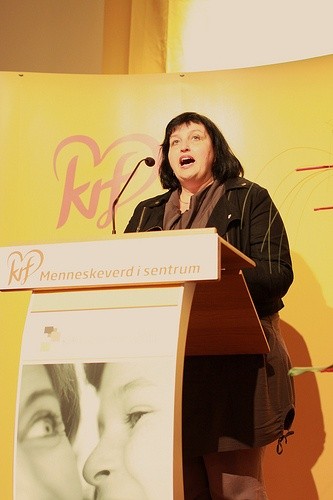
122 111 290 494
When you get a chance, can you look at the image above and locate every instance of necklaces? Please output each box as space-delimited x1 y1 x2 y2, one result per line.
179 198 190 205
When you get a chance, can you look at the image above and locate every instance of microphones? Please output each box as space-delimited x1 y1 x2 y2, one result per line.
111 158 155 233
